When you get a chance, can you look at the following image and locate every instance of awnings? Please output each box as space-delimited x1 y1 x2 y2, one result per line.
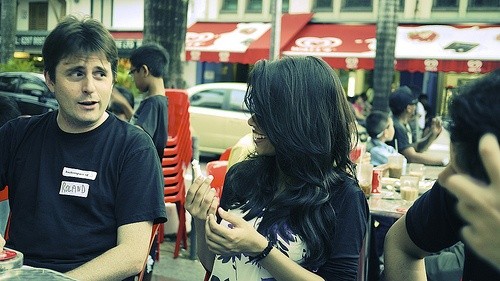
185 13 500 74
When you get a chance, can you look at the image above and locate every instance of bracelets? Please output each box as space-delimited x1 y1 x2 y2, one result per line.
248 241 275 262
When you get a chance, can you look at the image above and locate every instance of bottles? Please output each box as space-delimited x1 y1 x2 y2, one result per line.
358 151 373 198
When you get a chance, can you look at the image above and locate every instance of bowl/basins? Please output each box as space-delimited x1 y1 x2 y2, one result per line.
380 177 400 187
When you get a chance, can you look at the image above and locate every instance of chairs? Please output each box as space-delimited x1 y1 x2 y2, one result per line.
152 90 233 260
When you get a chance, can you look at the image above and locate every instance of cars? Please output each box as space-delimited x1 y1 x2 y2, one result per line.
0 70 59 116
186 81 255 160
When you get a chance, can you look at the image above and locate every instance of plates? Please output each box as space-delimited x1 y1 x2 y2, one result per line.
395 180 436 194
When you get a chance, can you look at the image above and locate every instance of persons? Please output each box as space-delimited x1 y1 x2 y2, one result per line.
0 13 168 280
343 86 450 258
384 68 500 281
185 56 369 281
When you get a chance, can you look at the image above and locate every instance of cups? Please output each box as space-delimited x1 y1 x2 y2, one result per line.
388 153 403 179
400 175 420 201
408 162 424 182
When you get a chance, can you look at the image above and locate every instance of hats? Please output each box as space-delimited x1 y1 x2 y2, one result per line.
389 85 418 112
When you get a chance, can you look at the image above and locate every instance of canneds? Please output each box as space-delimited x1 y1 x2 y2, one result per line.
372 169 381 193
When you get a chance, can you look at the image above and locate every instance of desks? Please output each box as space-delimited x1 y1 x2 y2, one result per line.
367 132 451 218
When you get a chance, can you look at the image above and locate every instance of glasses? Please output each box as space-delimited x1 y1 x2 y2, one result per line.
128 66 138 79
